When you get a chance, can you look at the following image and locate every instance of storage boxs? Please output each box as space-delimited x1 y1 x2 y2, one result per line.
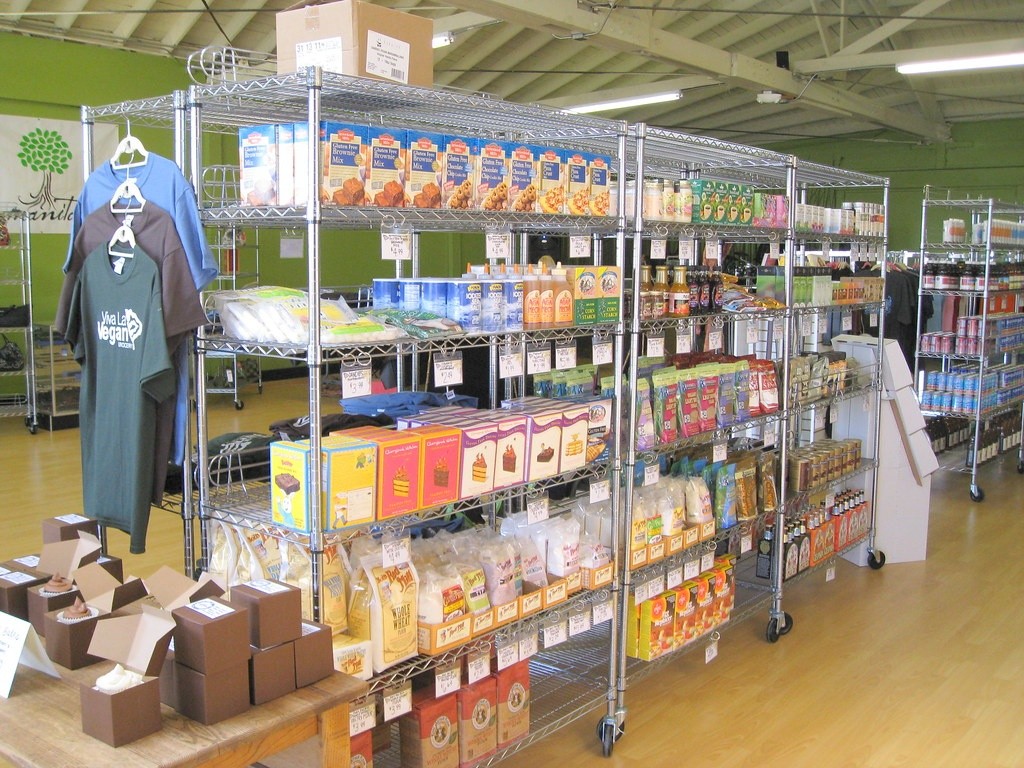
322 440 377 534
249 644 296 707
270 441 312 532
493 414 529 490
377 434 420 521
454 673 497 768
171 595 251 675
528 410 561 483
293 618 335 689
44 561 149 671
231 578 303 649
275 0 435 89
460 421 497 498
26 529 103 636
80 605 177 748
559 404 590 476
419 426 461 510
174 660 250 727
42 514 99 566
638 591 676 662
489 656 529 751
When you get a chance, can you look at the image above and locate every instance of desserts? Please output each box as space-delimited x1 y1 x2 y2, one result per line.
43 571 72 593
61 596 92 620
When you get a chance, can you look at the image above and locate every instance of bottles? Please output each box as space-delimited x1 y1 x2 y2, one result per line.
609 177 692 222
757 487 871 582
842 201 884 235
462 259 574 330
639 265 724 321
921 218 1024 291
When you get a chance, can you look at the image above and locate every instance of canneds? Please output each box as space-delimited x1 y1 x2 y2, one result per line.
943 219 1024 245
920 312 1024 356
842 201 885 237
920 362 1024 414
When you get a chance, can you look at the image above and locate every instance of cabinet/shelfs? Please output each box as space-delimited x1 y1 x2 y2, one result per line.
0 44 1024 768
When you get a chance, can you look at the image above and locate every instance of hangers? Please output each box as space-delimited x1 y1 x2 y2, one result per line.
111 179 145 212
108 215 136 258
109 121 147 169
